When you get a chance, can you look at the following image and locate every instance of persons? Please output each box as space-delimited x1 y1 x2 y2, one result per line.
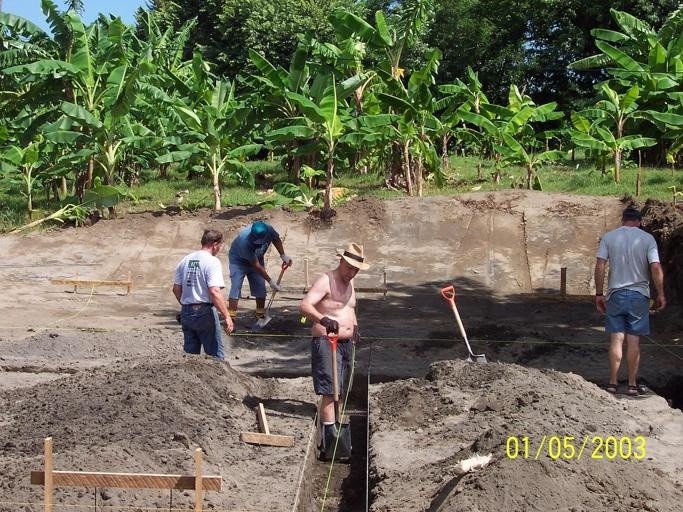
172 229 234 362
298 242 370 464
229 220 293 322
594 208 666 395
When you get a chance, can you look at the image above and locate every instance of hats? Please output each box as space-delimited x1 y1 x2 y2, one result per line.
334 242 372 271
249 221 269 246
623 209 643 220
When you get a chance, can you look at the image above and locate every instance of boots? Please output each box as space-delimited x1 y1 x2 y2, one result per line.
318 423 352 462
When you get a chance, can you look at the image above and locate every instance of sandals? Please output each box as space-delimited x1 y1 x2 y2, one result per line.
605 382 619 394
625 384 649 398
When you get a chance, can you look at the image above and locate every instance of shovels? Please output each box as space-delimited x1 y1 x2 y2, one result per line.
440 287 486 361
252 261 289 331
327 331 351 455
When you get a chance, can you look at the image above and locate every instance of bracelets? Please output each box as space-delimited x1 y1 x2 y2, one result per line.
596 292 603 296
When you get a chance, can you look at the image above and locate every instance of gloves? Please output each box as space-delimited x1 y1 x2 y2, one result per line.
350 324 364 346
320 317 341 335
267 280 282 292
281 255 293 267
